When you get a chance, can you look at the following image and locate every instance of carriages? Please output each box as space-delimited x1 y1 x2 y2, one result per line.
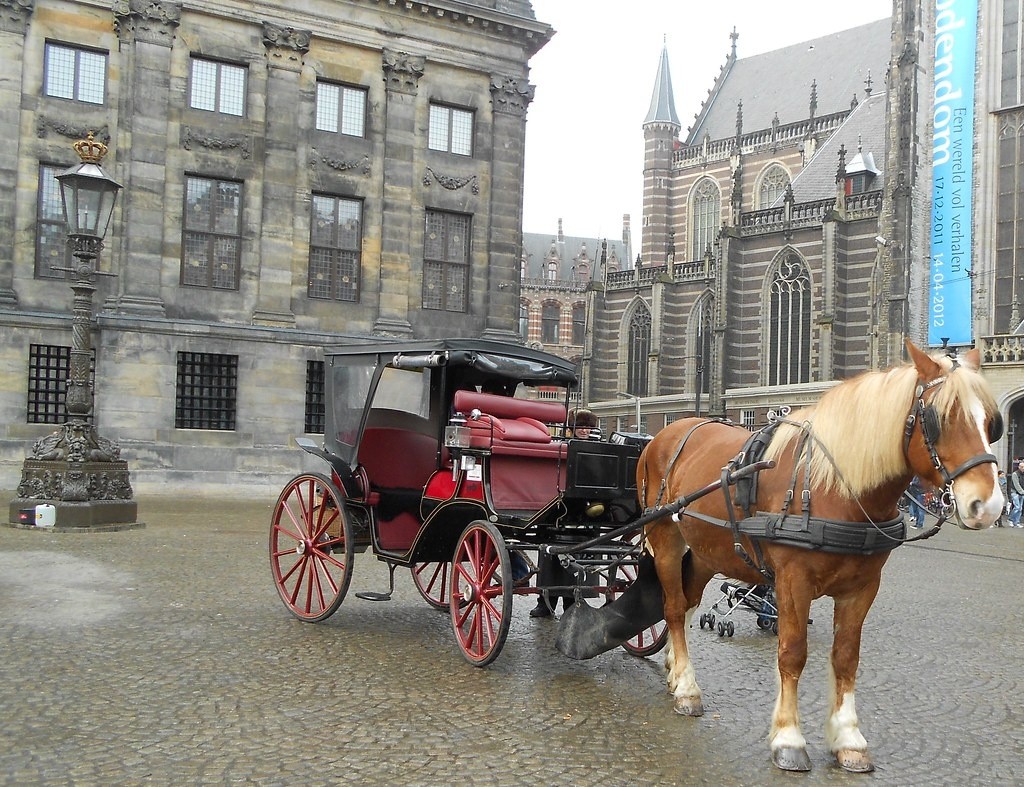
269 336 1007 772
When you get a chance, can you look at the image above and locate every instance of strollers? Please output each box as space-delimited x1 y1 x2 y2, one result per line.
700 579 779 637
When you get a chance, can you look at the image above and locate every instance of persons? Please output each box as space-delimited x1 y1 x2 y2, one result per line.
994 462 1024 529
530 407 598 617
905 473 926 529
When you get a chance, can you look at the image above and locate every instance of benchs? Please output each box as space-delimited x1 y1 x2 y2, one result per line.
455 391 602 511
335 427 451 551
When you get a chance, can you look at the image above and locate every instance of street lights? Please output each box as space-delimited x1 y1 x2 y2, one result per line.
8 131 138 528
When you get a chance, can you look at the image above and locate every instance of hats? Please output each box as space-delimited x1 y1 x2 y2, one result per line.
568 407 598 430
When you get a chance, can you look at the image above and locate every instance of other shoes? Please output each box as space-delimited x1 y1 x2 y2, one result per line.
910 524 923 530
1014 524 1023 528
998 525 1005 528
1006 520 1014 528
909 516 916 522
530 602 555 617
994 521 997 526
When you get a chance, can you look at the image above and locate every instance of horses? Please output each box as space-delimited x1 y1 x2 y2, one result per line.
635 335 1006 773
